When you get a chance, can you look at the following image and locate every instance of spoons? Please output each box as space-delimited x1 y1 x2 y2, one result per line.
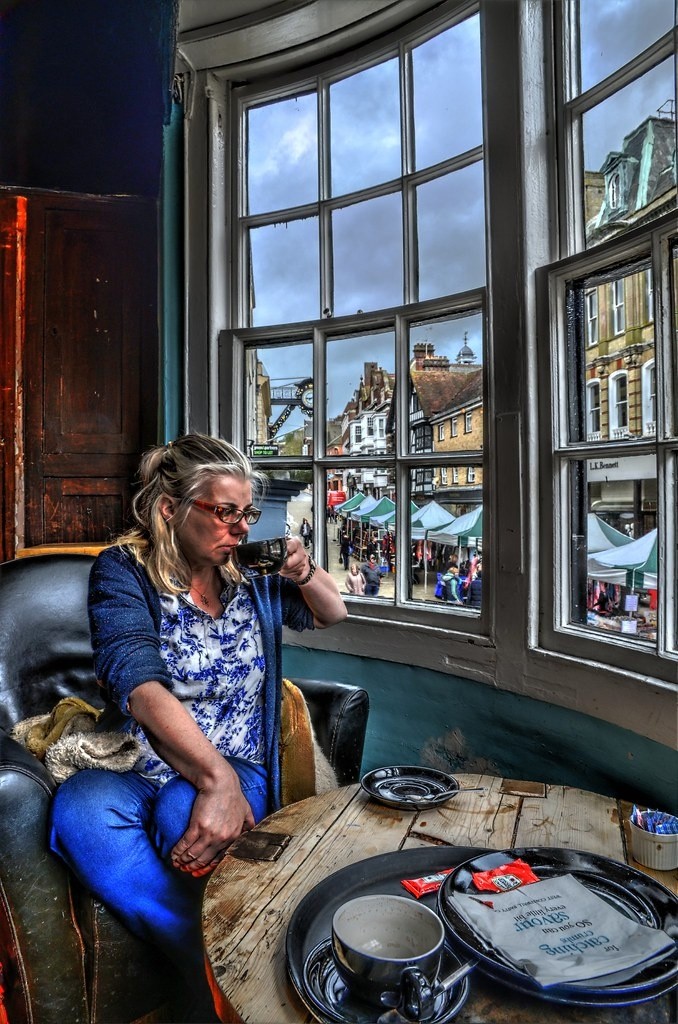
376 959 481 1023
405 787 484 803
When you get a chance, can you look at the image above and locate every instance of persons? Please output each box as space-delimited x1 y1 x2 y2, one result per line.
591 592 608 612
50 431 349 967
367 537 375 560
345 564 366 595
299 517 311 549
435 553 482 608
339 535 350 570
361 554 383 596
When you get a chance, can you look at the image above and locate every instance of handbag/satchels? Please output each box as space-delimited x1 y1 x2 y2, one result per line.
339 554 343 563
348 541 354 555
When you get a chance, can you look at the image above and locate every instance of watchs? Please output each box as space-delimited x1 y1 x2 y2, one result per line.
294 555 317 586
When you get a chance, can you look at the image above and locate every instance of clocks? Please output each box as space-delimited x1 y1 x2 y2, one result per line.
301 388 314 408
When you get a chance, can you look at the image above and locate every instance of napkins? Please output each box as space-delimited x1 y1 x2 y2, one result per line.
448 872 676 987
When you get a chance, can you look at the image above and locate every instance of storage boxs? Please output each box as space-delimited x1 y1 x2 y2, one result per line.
628 811 678 872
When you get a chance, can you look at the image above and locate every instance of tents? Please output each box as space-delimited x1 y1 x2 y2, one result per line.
331 493 364 548
369 499 417 553
587 512 636 554
341 494 376 551
427 503 483 567
587 527 659 619
351 496 395 565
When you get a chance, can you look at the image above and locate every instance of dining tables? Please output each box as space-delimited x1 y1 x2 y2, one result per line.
201 773 677 1024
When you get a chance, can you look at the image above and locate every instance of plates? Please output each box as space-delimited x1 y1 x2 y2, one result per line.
302 935 469 1024
360 764 459 811
438 844 677 1012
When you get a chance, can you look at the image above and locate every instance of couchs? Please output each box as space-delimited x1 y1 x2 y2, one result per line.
0 554 371 1023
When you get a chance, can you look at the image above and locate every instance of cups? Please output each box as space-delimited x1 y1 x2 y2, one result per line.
628 811 678 872
328 893 445 1024
232 538 287 580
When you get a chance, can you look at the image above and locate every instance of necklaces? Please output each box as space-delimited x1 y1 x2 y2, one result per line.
189 567 214 605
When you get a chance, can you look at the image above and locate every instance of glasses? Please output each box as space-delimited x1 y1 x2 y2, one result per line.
188 498 262 524
